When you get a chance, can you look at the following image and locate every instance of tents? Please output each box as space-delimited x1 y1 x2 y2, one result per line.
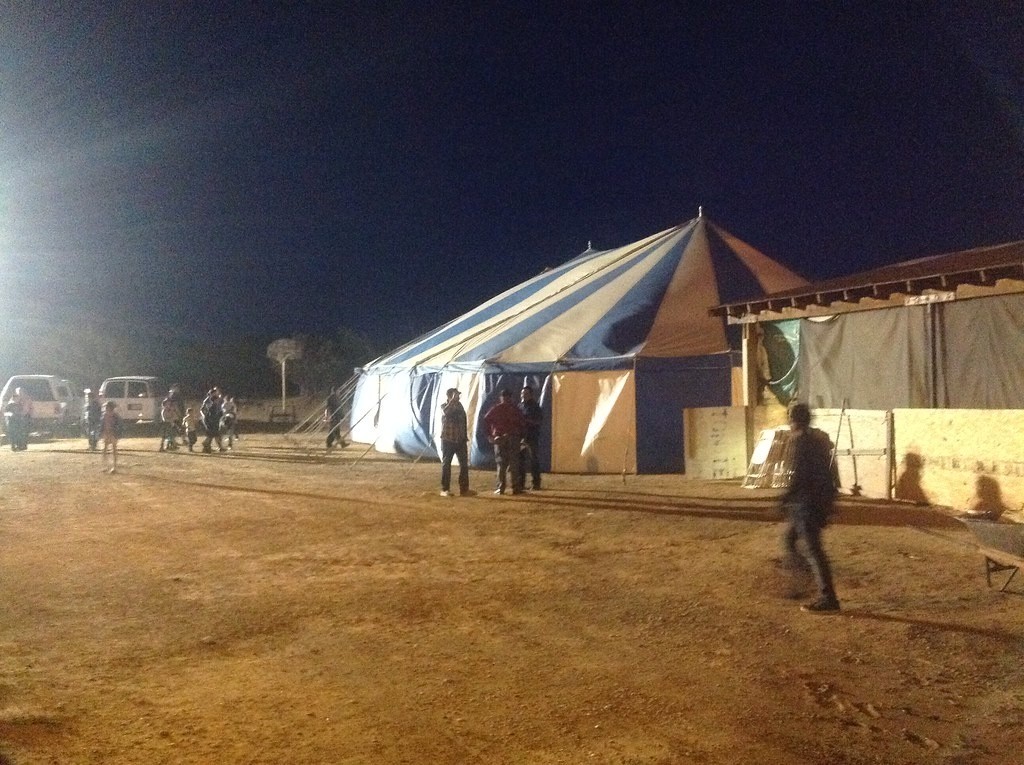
349 204 813 476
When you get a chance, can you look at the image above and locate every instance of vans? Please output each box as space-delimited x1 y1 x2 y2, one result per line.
98 376 169 424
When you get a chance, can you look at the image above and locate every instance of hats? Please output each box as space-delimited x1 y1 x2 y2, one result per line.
446 388 463 396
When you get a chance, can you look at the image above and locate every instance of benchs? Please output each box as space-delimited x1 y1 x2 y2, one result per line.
964 518 1024 591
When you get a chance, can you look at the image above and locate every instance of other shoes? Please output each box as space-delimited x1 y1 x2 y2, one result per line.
529 485 540 491
493 489 503 494
342 442 350 449
461 490 478 497
440 491 455 497
801 601 842 614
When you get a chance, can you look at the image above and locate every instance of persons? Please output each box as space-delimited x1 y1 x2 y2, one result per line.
439 388 478 497
517 386 545 490
483 387 527 494
324 386 350 448
3 382 239 474
770 405 840 615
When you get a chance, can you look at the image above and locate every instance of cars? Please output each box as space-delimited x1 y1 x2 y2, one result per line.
0 375 86 435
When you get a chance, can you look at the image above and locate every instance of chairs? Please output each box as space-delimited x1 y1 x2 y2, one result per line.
740 426 798 490
